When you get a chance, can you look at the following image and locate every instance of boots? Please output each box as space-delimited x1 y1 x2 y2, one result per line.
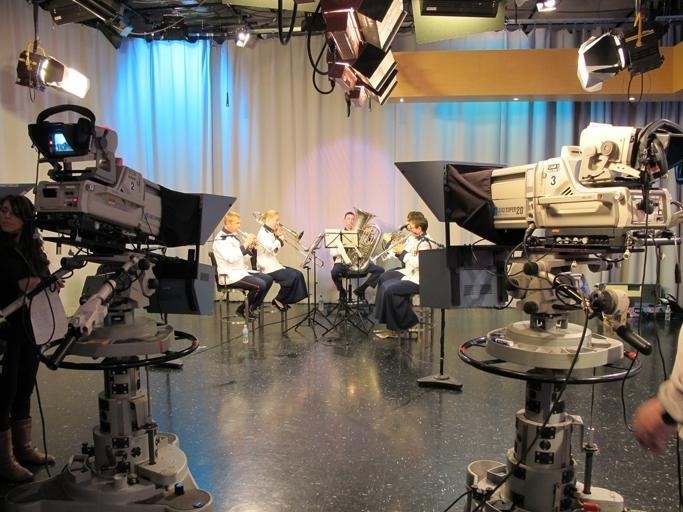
8 415 56 466
0 427 34 482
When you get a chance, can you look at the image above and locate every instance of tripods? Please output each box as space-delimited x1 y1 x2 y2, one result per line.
346 259 375 333
282 268 328 338
295 252 336 332
326 251 356 328
322 264 368 346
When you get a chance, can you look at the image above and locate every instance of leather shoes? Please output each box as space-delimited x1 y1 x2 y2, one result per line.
353 289 364 300
236 305 256 320
271 300 286 311
339 290 346 304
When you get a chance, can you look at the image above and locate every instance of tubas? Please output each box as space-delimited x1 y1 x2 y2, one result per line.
341 207 382 273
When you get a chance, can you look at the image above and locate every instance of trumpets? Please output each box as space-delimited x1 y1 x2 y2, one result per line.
238 230 278 257
369 232 414 266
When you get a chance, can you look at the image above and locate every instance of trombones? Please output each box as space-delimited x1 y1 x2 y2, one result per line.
253 211 324 267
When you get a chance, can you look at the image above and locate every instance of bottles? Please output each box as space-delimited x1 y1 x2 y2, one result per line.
318 294 324 311
665 305 671 320
242 325 249 343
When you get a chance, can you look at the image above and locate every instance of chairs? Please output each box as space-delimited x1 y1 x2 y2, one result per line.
208 252 256 346
407 292 435 342
249 256 288 326
325 270 368 318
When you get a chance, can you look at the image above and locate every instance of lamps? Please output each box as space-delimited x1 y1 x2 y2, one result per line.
577 0 667 93
15 1 90 98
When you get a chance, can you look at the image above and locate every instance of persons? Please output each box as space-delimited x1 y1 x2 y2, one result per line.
633 323 683 453
0 194 66 482
330 212 385 301
381 211 431 272
372 216 437 340
255 209 309 312
212 211 273 321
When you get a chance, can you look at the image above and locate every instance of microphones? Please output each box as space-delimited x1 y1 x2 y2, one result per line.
398 223 410 230
221 232 237 237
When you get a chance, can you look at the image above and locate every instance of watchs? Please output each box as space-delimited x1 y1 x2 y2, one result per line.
660 409 676 425
